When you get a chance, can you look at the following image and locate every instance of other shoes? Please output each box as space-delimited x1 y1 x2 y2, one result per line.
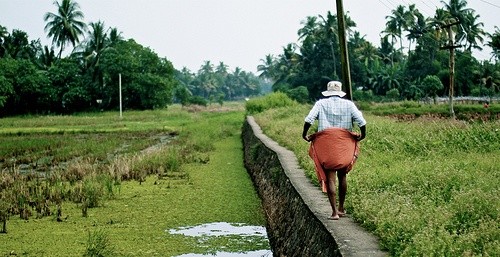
337 210 345 216
327 214 339 219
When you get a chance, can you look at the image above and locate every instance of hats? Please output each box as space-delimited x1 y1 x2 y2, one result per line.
321 81 346 97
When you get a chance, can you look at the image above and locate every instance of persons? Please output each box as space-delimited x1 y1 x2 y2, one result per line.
300 81 366 219
